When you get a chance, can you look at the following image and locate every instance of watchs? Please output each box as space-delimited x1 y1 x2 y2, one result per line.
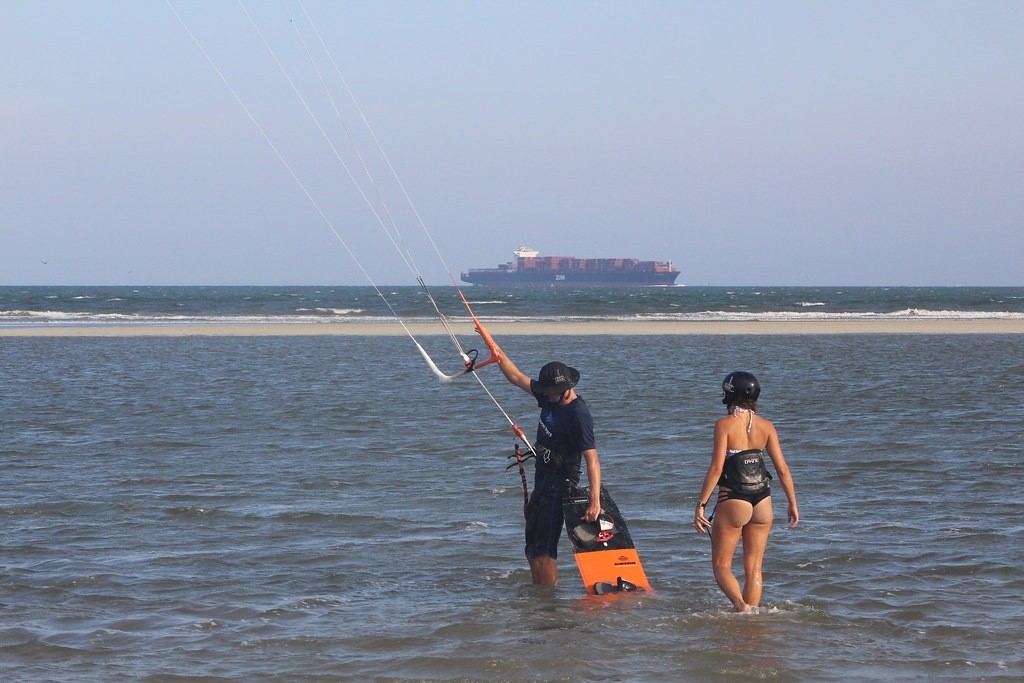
696 500 706 507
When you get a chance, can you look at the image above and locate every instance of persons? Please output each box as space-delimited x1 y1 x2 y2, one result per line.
474 320 600 585
693 371 798 613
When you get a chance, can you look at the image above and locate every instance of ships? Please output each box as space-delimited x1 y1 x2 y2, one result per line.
460 242 681 286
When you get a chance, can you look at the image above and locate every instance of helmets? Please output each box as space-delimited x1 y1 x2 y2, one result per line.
722 371 760 404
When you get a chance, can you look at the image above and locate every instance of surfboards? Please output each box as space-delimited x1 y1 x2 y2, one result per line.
561 465 652 598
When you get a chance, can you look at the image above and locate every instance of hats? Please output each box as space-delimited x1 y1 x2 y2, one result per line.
533 361 580 393
